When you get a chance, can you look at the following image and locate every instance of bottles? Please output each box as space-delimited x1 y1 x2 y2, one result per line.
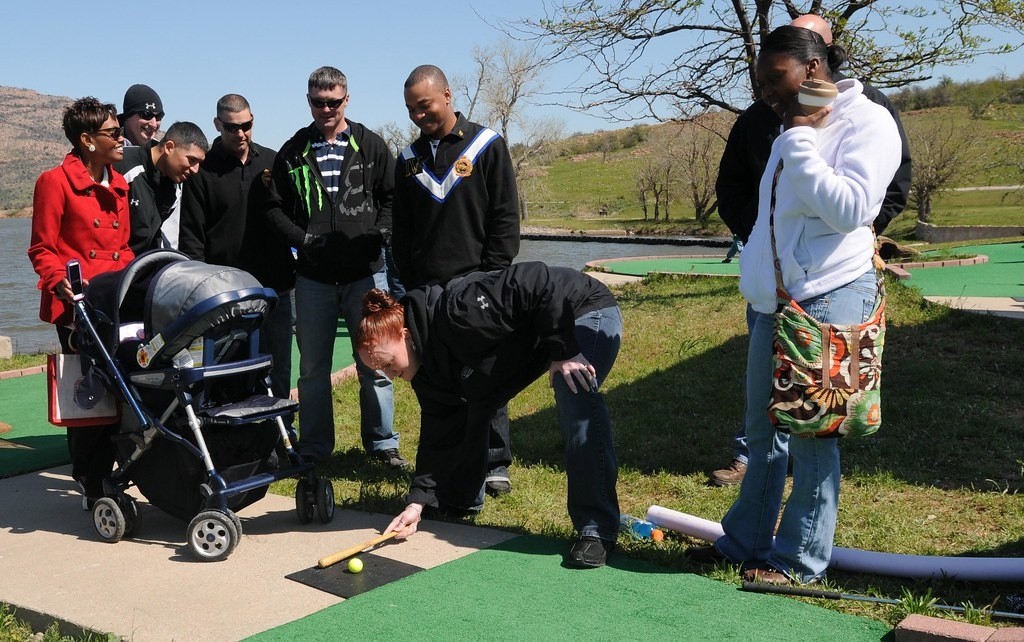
620 514 663 541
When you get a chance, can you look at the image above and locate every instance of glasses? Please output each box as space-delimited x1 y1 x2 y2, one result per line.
217 114 254 133
309 94 346 109
139 110 164 121
89 126 124 139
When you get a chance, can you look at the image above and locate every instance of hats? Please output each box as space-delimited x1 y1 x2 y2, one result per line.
124 84 163 116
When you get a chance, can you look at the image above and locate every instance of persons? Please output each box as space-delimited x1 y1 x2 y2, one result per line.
722 232 744 263
26 64 522 497
355 260 624 567
711 14 912 486
685 27 905 583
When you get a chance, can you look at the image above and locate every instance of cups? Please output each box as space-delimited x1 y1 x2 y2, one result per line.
798 78 839 127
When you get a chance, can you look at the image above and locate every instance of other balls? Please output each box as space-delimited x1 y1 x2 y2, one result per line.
348 558 363 573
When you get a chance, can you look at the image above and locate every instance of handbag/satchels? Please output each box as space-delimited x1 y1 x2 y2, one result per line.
47 353 119 427
767 288 886 438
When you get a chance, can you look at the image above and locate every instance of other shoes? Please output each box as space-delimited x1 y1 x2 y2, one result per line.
567 536 615 567
710 460 748 486
422 494 484 515
686 546 727 566
366 447 409 470
303 455 315 463
744 567 825 586
87 496 100 509
486 467 511 497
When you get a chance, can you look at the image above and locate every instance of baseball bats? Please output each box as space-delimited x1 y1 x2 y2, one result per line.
318 526 410 568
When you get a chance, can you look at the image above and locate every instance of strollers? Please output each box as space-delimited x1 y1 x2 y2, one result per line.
64 229 336 560
